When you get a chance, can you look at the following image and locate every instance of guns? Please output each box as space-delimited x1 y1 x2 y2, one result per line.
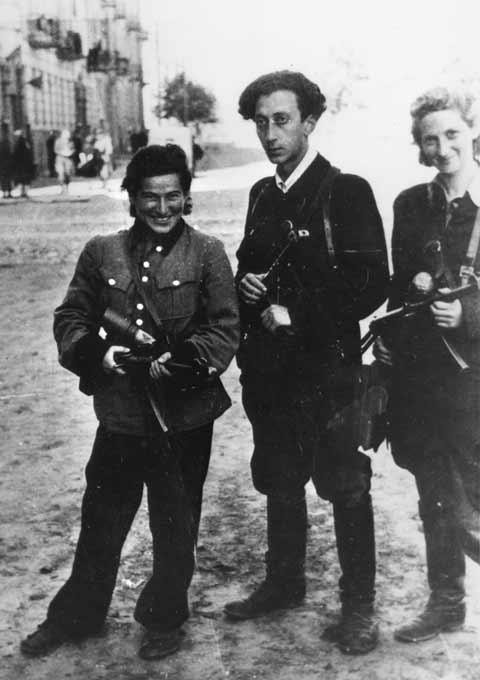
371 280 479 380
79 351 219 434
237 228 310 351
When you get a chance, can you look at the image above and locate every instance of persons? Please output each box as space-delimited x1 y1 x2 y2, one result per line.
0 119 204 199
373 90 480 644
223 69 389 652
21 142 240 661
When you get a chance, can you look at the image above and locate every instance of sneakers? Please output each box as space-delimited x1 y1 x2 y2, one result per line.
336 600 378 655
20 613 108 656
394 595 467 642
139 621 186 659
222 579 305 619
